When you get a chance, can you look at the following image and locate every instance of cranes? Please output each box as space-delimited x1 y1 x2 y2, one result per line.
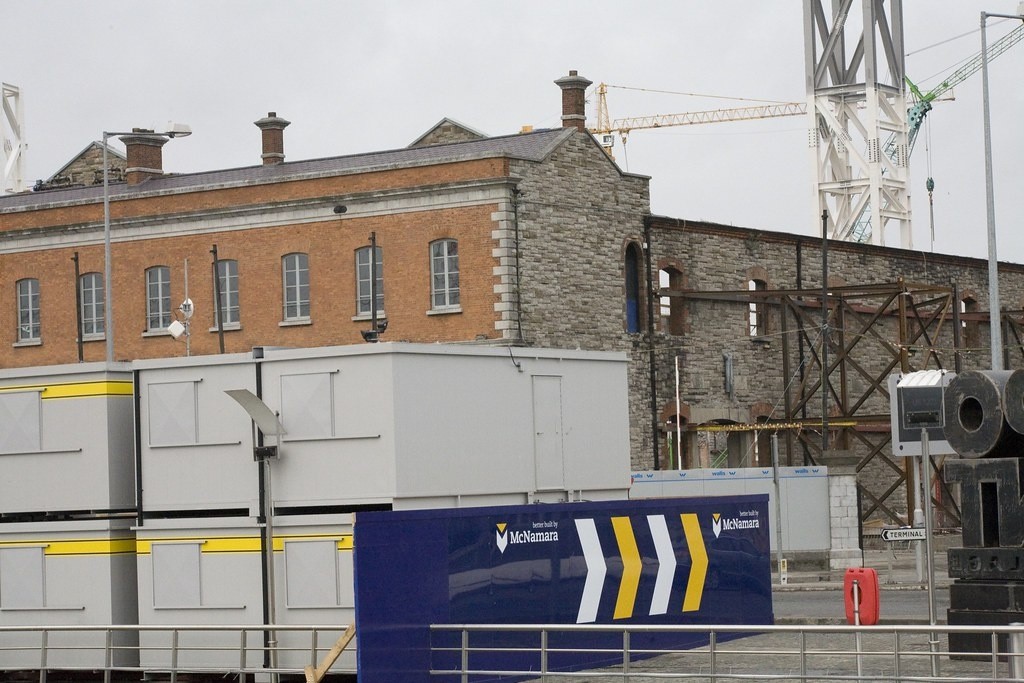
514 83 810 161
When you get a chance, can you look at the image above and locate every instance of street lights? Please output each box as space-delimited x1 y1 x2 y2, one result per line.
98 122 195 363
